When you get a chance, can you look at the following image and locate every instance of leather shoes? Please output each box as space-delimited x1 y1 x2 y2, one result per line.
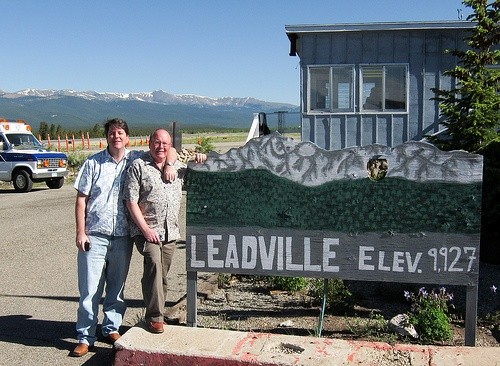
148 320 165 333
107 334 121 343
72 343 95 357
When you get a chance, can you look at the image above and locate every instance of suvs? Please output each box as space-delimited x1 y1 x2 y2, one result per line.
0 117 69 193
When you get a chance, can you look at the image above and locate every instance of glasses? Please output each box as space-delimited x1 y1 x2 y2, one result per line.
151 141 173 148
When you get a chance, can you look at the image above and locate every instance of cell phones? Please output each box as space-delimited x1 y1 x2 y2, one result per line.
84 242 91 251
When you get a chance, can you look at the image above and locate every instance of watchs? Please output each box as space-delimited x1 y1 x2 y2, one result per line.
166 161 174 166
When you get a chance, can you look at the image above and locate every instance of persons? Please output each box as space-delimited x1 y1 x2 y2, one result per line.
122 128 207 333
73 118 178 357
